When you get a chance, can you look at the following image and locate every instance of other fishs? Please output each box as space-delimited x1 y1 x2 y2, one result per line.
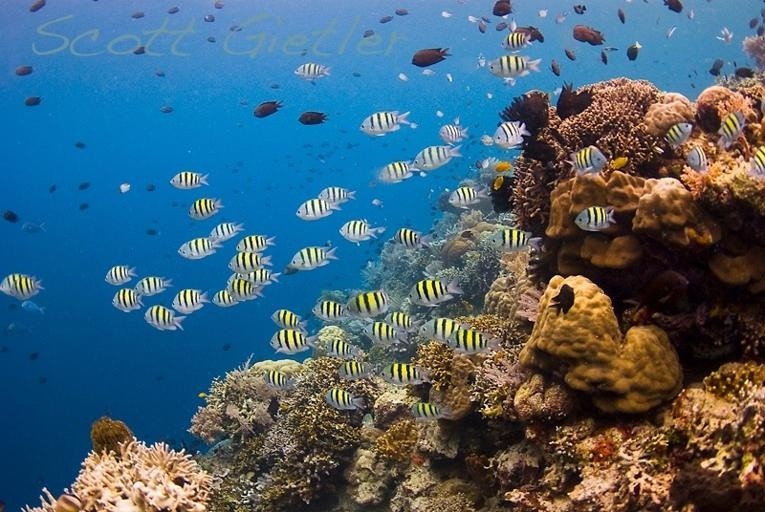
0 0 765 421
